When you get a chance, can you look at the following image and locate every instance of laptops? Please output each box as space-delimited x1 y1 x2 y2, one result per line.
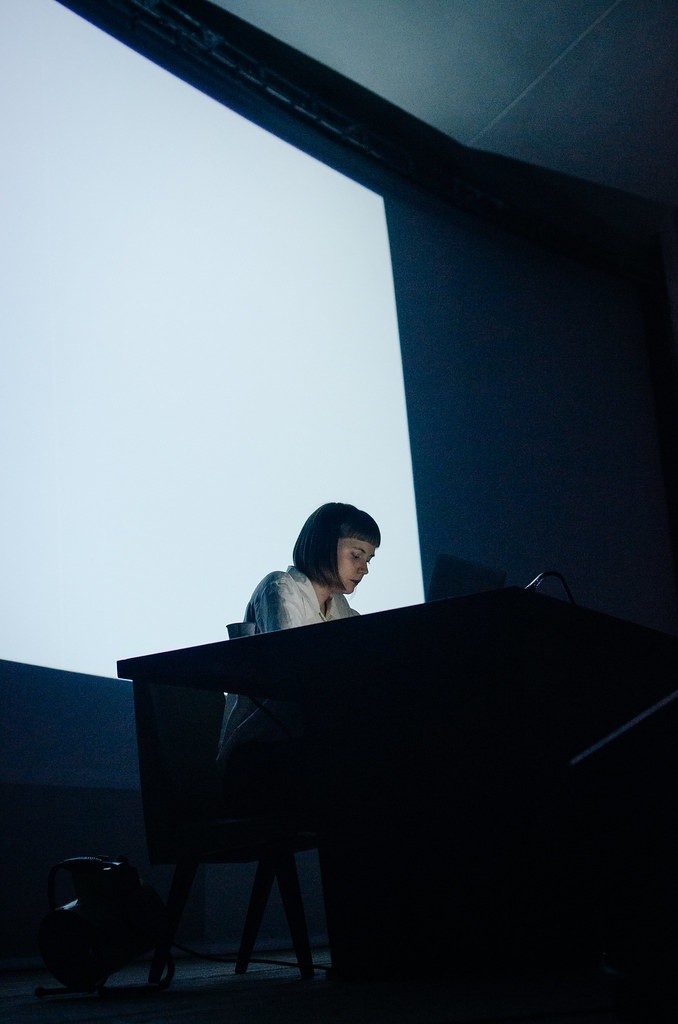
428 552 507 603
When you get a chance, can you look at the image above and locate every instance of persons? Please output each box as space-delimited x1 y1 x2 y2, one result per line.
217 502 443 824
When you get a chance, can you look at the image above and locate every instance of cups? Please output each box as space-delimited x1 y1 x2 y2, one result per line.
226 622 256 639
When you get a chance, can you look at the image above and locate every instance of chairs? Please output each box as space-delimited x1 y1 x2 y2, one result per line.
134 680 319 988
